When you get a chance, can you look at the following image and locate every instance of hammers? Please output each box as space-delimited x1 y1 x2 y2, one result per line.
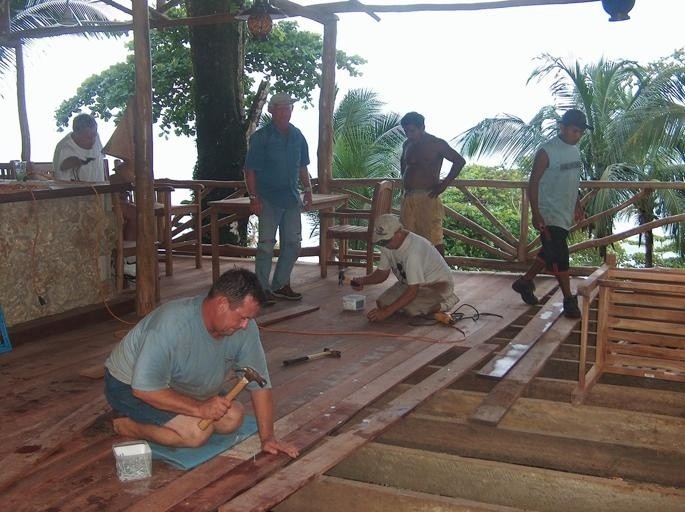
283 347 342 366
197 367 267 431
338 269 360 288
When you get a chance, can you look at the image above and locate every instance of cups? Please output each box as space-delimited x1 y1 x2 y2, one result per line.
14 161 27 181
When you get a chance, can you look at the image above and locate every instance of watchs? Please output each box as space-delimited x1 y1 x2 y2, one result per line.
52 113 109 182
249 193 260 200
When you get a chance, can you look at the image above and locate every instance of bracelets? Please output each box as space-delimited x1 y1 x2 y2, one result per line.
302 185 312 194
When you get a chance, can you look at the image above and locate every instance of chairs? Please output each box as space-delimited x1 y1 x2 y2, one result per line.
120 183 204 276
317 180 395 276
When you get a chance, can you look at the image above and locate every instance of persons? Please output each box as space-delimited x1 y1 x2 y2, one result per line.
350 214 458 322
243 92 315 306
510 107 597 317
397 112 466 259
79 268 303 458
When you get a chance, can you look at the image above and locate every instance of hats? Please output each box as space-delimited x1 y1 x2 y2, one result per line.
562 107 595 132
371 212 401 243
267 91 297 108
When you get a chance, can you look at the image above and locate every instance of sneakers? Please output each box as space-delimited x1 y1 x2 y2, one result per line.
96 408 127 434
272 285 302 300
261 292 277 307
511 275 540 305
441 292 461 310
563 295 583 319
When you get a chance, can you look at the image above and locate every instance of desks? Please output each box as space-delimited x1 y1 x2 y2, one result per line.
207 193 349 287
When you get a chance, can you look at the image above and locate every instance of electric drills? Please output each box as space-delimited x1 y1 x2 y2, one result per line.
425 312 455 324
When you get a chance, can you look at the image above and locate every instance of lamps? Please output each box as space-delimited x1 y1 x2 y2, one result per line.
234 1 288 43
